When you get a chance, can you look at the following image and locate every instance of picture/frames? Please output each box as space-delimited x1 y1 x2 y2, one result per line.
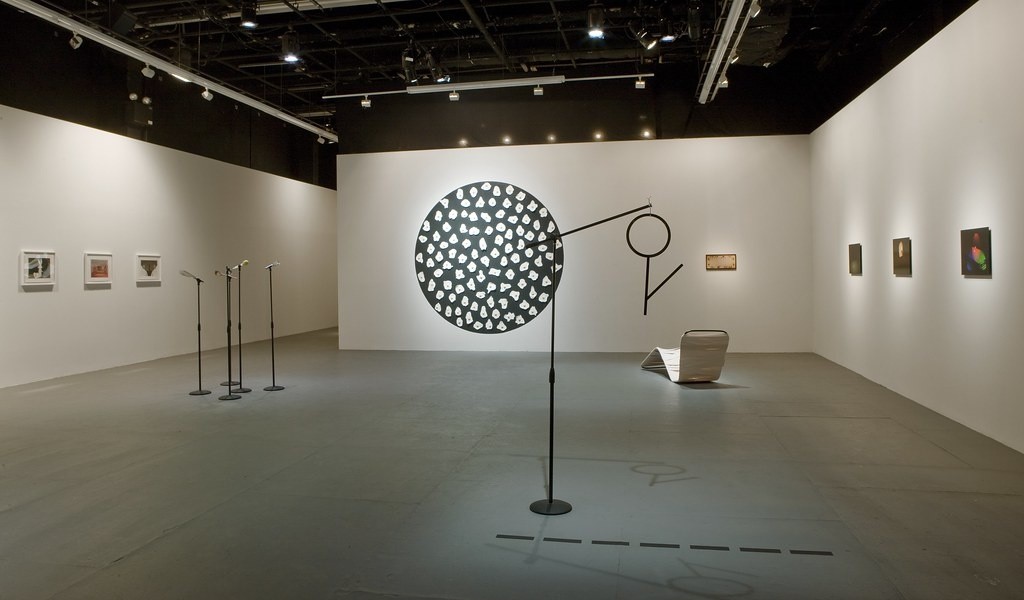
83 253 112 284
705 253 736 270
135 254 163 284
20 251 56 286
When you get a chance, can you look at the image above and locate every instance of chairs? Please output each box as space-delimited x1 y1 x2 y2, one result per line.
640 327 731 383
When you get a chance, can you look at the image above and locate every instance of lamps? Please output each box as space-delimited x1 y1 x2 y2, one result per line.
239 6 258 29
448 89 460 101
577 10 678 57
128 93 153 106
362 95 372 108
277 29 303 63
533 83 543 96
635 75 647 91
142 62 155 78
715 5 765 88
202 86 213 101
70 31 81 49
317 134 325 146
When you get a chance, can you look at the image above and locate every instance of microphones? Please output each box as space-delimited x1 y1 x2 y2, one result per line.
265 260 279 269
232 260 248 270
